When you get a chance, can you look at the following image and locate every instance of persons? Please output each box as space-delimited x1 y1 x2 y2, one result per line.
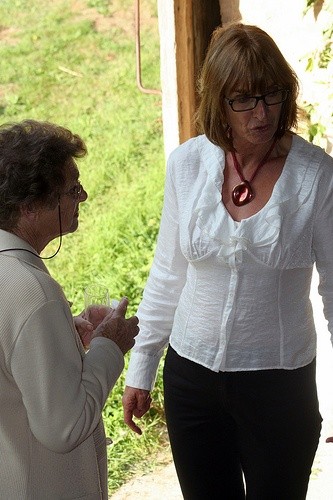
0 119 140 500
119 22 333 499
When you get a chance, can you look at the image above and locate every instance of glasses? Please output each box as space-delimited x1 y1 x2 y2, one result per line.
44 182 84 201
221 82 292 113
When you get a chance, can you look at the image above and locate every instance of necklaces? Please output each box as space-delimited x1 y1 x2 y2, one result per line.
228 128 279 208
